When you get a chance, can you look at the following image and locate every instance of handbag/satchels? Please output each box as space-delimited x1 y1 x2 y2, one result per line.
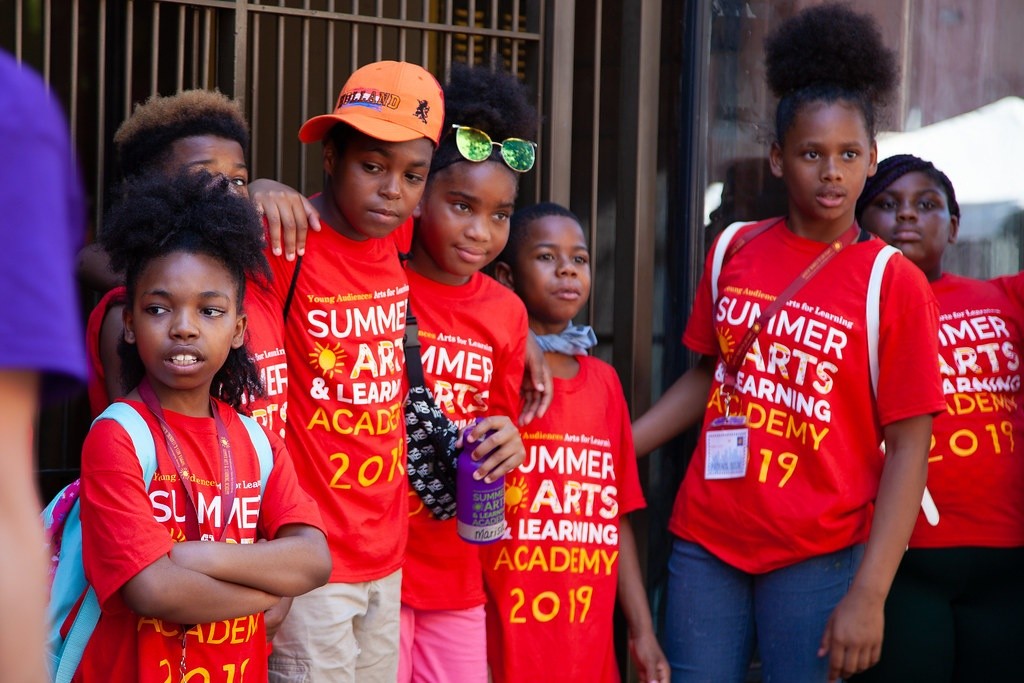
405 387 466 521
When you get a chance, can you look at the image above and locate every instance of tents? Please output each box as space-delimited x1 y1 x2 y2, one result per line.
704 97 1024 245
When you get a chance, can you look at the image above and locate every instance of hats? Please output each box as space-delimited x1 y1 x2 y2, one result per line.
298 61 445 151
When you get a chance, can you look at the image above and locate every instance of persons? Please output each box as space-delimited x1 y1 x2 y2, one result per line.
483 199 671 683
852 154 1024 683
395 64 529 683
0 56 331 683
626 7 946 683
250 62 554 683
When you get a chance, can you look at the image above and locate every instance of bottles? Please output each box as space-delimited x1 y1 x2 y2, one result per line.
457 418 505 542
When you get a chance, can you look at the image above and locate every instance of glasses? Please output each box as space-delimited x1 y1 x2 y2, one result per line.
441 124 537 173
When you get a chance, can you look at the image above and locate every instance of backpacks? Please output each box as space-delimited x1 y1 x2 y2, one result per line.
40 402 275 683
